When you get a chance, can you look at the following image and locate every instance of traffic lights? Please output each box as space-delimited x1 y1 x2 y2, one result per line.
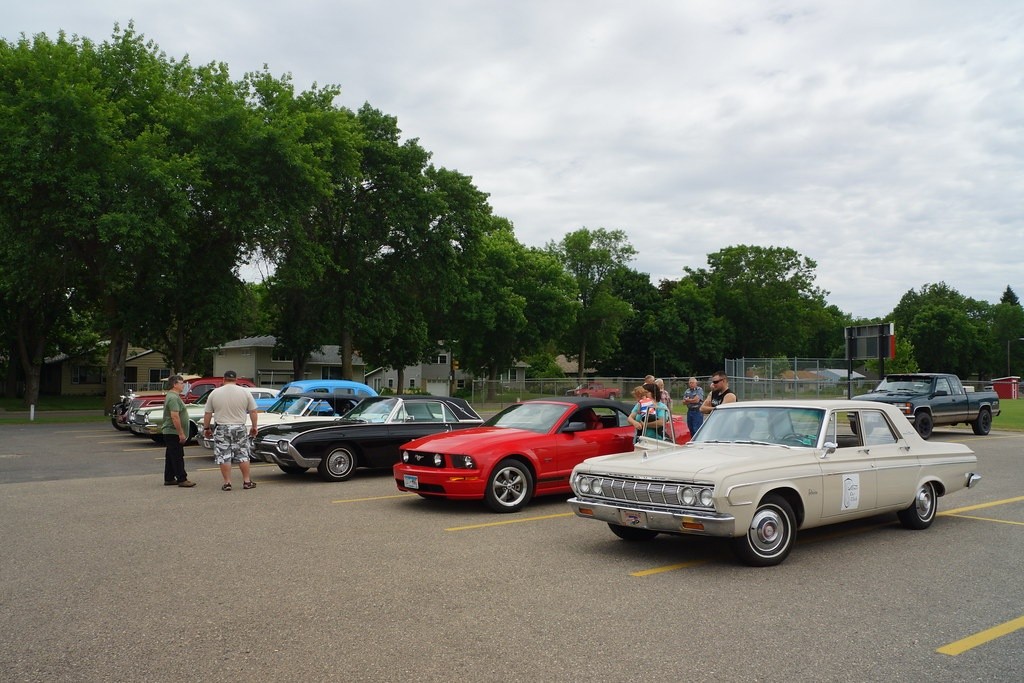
453 361 459 370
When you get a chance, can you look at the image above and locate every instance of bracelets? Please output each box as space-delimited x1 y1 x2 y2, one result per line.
204 427 209 430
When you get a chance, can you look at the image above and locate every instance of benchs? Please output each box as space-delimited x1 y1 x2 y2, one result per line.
816 435 877 448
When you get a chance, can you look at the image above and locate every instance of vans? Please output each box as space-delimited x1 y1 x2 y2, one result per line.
983 386 992 392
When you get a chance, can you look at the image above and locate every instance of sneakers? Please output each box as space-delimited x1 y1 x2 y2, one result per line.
222 483 231 491
243 480 256 489
164 479 178 485
179 481 196 487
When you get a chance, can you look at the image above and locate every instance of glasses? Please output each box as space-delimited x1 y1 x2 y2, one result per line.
712 378 726 384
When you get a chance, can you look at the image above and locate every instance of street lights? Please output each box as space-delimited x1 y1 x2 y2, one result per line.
1008 338 1024 377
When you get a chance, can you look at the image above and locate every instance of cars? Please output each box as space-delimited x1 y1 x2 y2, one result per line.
565 399 982 568
108 374 259 438
195 393 409 463
250 396 485 483
392 397 691 513
255 379 388 416
128 387 282 443
564 383 588 398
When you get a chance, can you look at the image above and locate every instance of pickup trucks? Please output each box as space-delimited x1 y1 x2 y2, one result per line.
574 382 622 400
848 372 1000 438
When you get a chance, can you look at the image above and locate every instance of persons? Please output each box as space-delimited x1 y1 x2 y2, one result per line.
682 377 703 438
627 375 673 445
699 373 737 436
202 371 258 491
161 376 197 488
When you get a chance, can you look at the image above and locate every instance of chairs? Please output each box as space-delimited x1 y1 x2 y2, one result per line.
737 418 754 435
582 408 603 429
